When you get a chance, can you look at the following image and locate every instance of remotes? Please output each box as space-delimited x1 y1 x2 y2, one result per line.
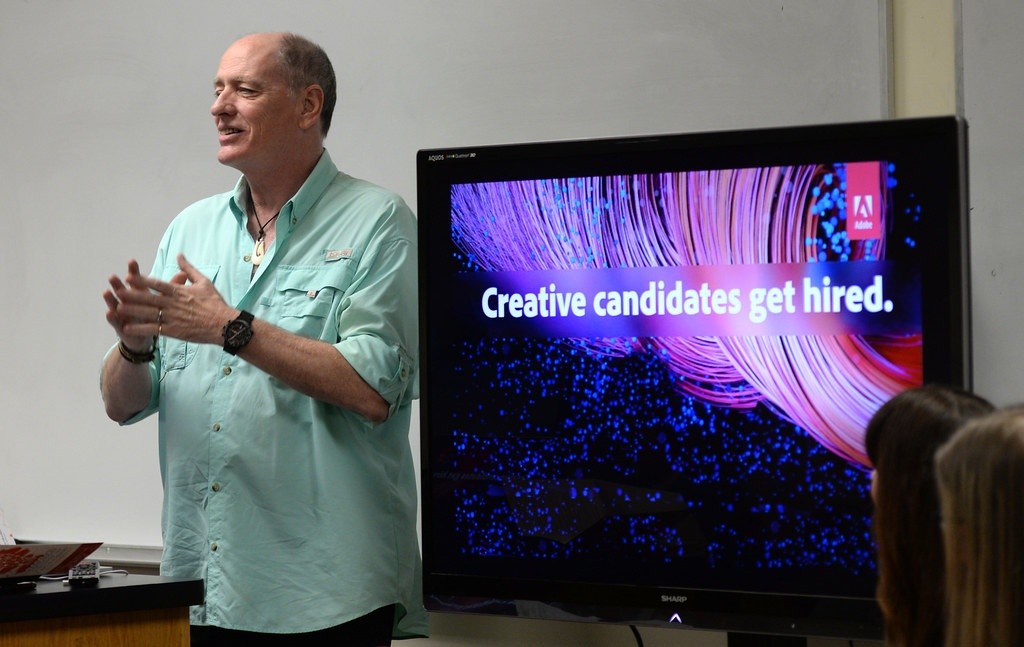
69 561 100 588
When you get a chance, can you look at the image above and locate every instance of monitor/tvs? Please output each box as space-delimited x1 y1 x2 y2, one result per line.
416 114 974 642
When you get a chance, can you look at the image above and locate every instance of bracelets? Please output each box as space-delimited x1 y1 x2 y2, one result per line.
118 335 158 364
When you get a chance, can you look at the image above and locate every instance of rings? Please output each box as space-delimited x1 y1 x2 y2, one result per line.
158 322 162 335
156 308 162 323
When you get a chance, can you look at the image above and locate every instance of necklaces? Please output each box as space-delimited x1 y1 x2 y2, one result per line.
250 198 280 266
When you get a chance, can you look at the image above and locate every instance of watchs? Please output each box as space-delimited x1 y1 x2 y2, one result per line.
221 310 255 357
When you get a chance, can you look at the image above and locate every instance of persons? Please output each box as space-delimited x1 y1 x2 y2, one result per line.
98 33 430 647
935 403 1024 647
863 385 998 647
